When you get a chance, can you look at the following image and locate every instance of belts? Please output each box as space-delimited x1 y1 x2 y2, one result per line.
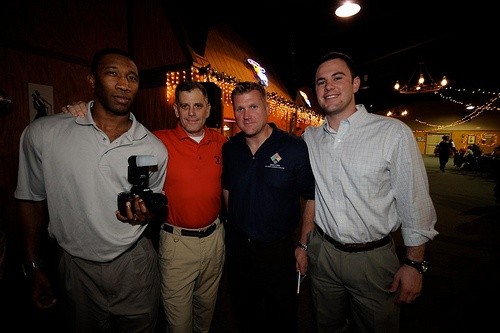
314 222 391 253
162 216 224 238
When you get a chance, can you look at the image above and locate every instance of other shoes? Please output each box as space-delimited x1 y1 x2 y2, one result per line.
438 165 446 173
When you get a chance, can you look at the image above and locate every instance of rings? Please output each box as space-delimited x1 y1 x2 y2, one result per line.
67 105 72 108
133 213 137 220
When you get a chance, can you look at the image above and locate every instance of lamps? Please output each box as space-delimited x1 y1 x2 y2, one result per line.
335 0 361 17
393 62 448 94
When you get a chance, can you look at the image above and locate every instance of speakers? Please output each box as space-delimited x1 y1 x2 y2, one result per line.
197 81 221 129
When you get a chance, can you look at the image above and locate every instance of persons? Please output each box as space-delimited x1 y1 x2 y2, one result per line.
434 135 500 203
221 83 316 333
13 48 170 333
299 52 439 333
62 81 229 333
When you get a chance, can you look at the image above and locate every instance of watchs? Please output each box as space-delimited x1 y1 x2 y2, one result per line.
404 258 429 274
296 240 308 251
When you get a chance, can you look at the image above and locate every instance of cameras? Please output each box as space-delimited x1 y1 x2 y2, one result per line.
116 154 168 217
